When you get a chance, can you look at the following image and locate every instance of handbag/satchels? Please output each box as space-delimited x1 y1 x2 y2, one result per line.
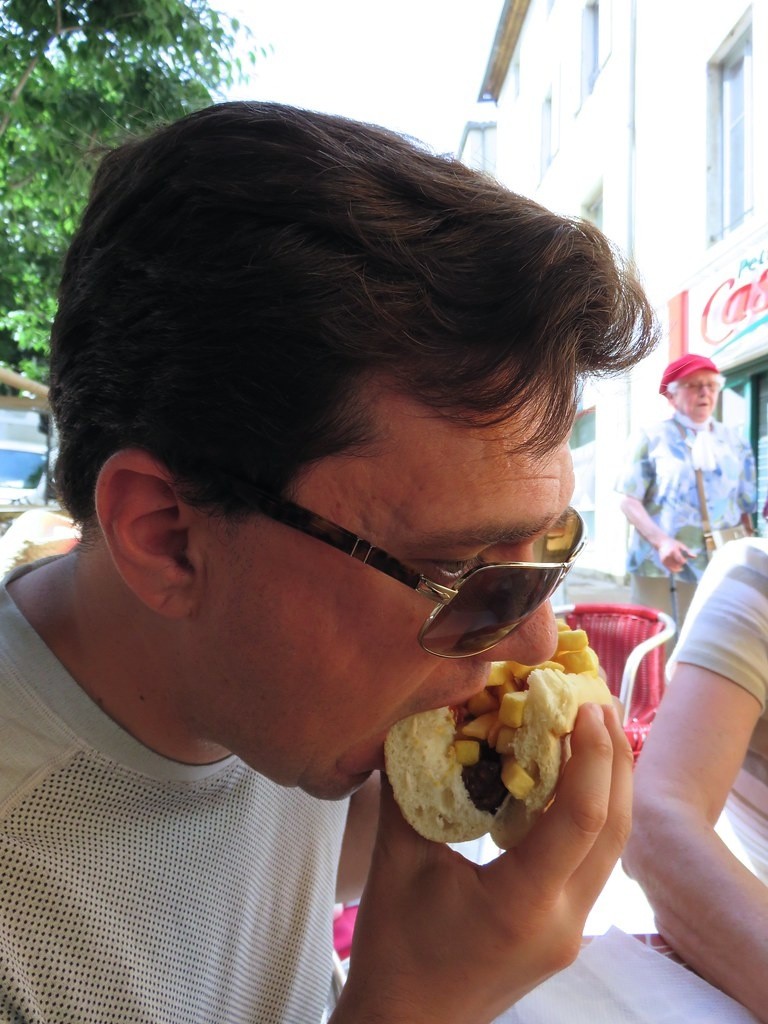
705 523 753 562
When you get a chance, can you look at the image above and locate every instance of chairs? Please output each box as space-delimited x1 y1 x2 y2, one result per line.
554 604 677 766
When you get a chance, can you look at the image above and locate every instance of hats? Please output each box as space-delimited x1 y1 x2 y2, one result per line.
659 355 719 400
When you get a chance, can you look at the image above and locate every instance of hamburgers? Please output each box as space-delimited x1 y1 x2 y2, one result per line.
380 616 614 853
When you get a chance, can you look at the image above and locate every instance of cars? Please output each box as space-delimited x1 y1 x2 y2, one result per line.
0 408 57 511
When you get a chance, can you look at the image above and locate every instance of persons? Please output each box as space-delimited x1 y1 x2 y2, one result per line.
618 536 768 1024
0 102 655 1024
615 355 758 652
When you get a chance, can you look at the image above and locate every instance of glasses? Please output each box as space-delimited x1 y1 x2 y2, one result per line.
677 380 720 392
211 470 587 658
